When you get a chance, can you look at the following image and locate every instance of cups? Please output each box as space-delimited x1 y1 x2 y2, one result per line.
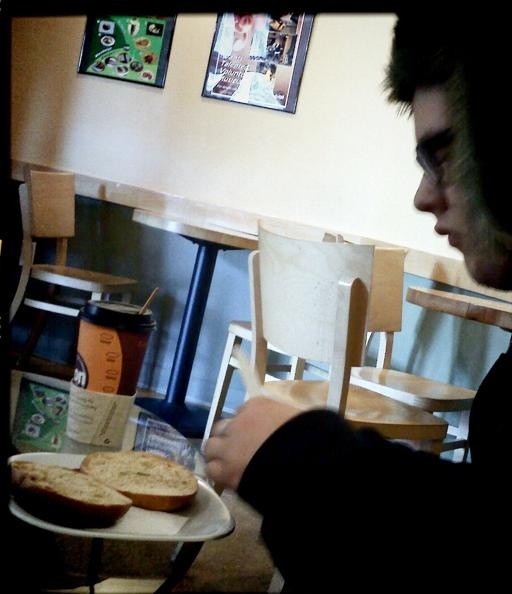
67 303 157 447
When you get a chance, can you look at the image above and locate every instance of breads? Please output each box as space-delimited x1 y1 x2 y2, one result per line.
9 459 133 530
79 449 199 510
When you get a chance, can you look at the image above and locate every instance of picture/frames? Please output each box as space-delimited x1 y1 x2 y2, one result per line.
201 14 315 114
77 15 178 88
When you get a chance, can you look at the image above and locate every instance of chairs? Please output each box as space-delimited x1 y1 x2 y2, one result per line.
200 234 335 448
8 170 140 369
339 236 476 465
156 221 448 594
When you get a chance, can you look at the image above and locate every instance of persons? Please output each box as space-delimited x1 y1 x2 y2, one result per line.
203 11 512 593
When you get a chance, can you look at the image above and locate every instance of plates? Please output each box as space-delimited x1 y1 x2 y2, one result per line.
4 450 234 543
